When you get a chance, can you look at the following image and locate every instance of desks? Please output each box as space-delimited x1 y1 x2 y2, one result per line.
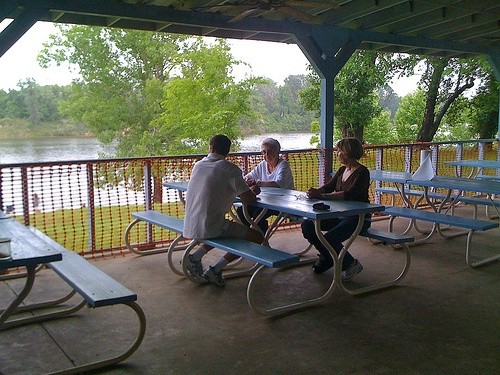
444 160 500 182
367 170 500 268
0 216 63 325
162 181 385 313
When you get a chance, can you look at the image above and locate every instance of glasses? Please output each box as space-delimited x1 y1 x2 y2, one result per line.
261 151 275 155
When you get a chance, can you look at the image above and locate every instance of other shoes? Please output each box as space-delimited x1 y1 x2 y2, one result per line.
312 254 334 274
342 259 363 282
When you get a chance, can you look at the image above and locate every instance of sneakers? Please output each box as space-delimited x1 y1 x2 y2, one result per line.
202 265 225 288
179 254 203 278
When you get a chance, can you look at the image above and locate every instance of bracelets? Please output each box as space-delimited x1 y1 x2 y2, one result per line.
257 180 262 186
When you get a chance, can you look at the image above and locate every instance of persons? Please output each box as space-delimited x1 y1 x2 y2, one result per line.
179 135 263 288
236 138 295 235
301 137 372 280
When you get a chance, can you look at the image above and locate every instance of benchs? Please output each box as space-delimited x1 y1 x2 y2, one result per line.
24 175 500 375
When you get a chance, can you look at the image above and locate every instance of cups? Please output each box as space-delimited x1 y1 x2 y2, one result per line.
0 238 12 261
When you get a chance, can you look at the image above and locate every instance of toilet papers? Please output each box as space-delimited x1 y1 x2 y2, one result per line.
411 150 436 182
